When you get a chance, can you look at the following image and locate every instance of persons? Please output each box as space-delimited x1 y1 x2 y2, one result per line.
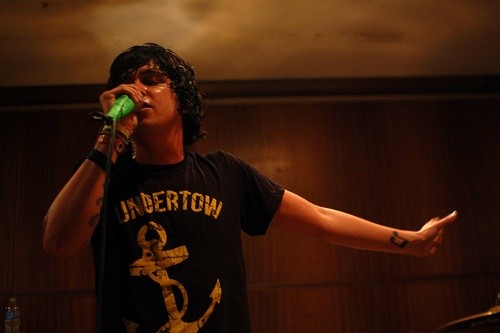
41 41 458 333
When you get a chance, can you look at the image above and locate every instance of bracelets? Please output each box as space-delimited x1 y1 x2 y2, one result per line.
85 122 137 175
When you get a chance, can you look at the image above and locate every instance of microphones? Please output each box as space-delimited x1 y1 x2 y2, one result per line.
105 93 144 126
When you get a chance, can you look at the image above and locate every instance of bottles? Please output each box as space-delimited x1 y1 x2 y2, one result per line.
4 298 20 333
